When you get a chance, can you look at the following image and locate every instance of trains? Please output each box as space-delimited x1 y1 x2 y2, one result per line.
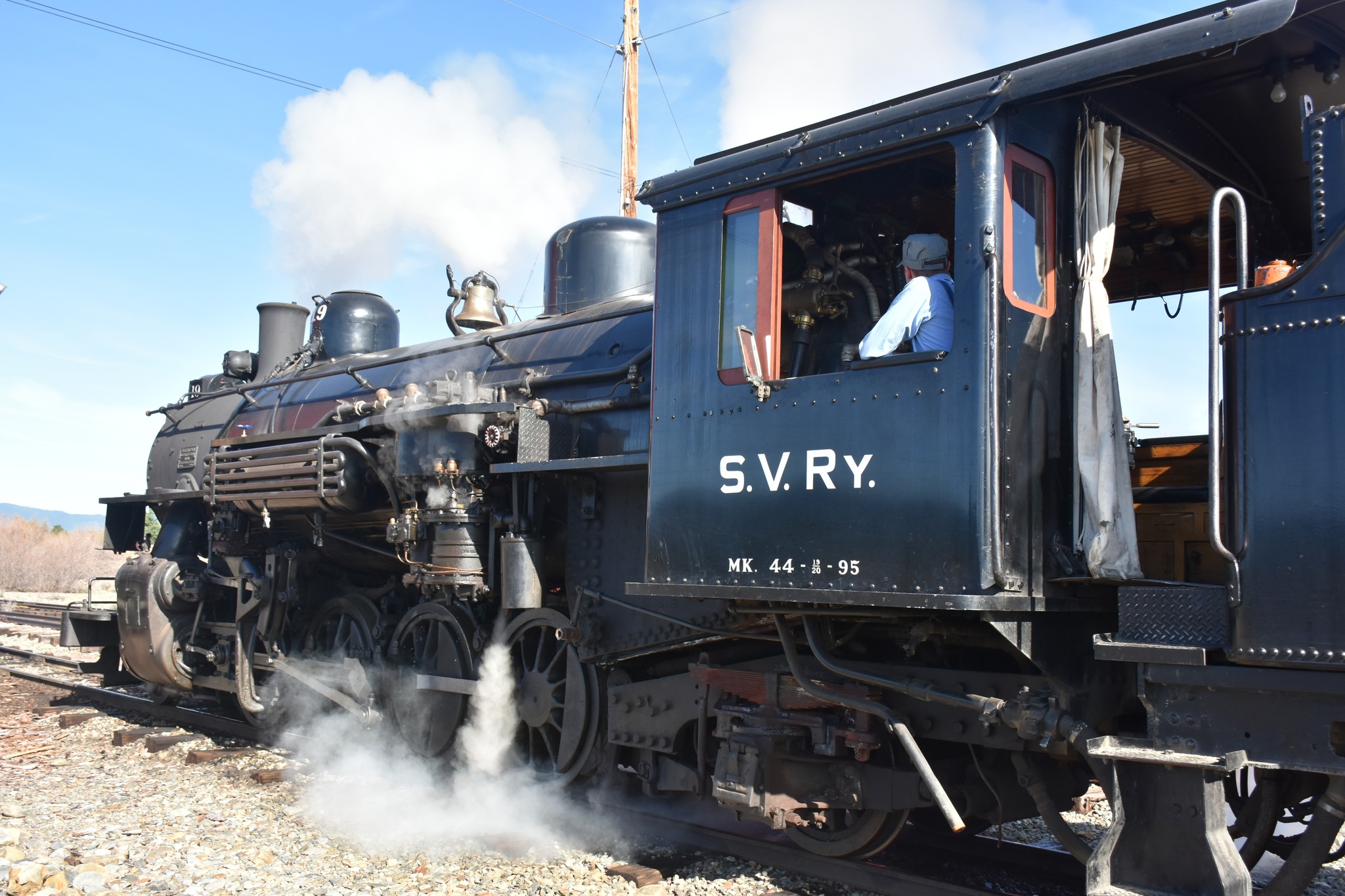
60 0 1345 896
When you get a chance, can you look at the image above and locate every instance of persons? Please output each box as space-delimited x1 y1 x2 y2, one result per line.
858 233 954 360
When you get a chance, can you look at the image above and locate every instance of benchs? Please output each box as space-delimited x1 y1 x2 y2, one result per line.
1130 434 1227 583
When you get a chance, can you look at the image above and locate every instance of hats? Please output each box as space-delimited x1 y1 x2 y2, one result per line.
896 233 950 271
